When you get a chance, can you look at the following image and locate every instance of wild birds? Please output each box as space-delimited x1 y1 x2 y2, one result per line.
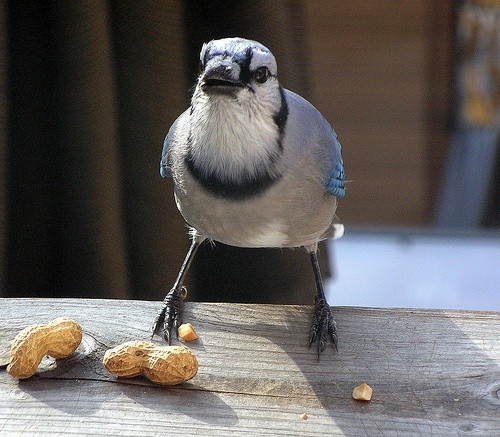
144 37 349 364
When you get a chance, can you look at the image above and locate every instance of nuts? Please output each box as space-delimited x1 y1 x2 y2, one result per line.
5 316 83 380
102 340 198 385
